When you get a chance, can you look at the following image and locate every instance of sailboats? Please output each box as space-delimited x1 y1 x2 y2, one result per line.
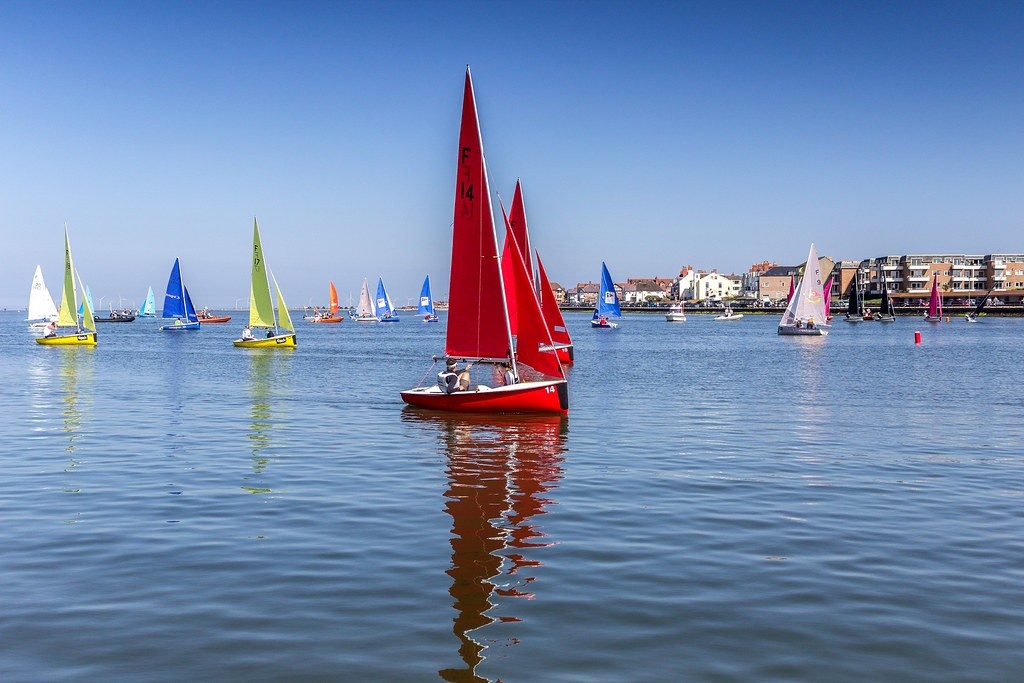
373 277 400 322
316 281 343 322
392 64 573 415
34 222 97 346
232 216 297 348
776 241 834 336
136 286 158 318
24 265 61 332
921 271 944 322
841 269 897 322
414 274 435 315
158 255 200 331
78 287 93 319
961 274 1005 322
502 177 573 367
589 261 621 328
352 278 380 323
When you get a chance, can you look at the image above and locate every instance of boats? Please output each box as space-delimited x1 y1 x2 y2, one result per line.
87 307 135 322
664 302 687 321
715 305 743 322
198 316 232 324
423 309 439 322
302 308 323 320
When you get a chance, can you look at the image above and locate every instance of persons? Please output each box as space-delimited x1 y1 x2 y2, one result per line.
841 305 980 324
201 312 214 320
600 315 611 327
793 316 819 331
313 306 391 320
425 313 432 321
43 322 59 339
174 317 185 326
241 326 272 341
502 362 516 385
491 362 510 388
437 358 474 395
109 308 135 320
